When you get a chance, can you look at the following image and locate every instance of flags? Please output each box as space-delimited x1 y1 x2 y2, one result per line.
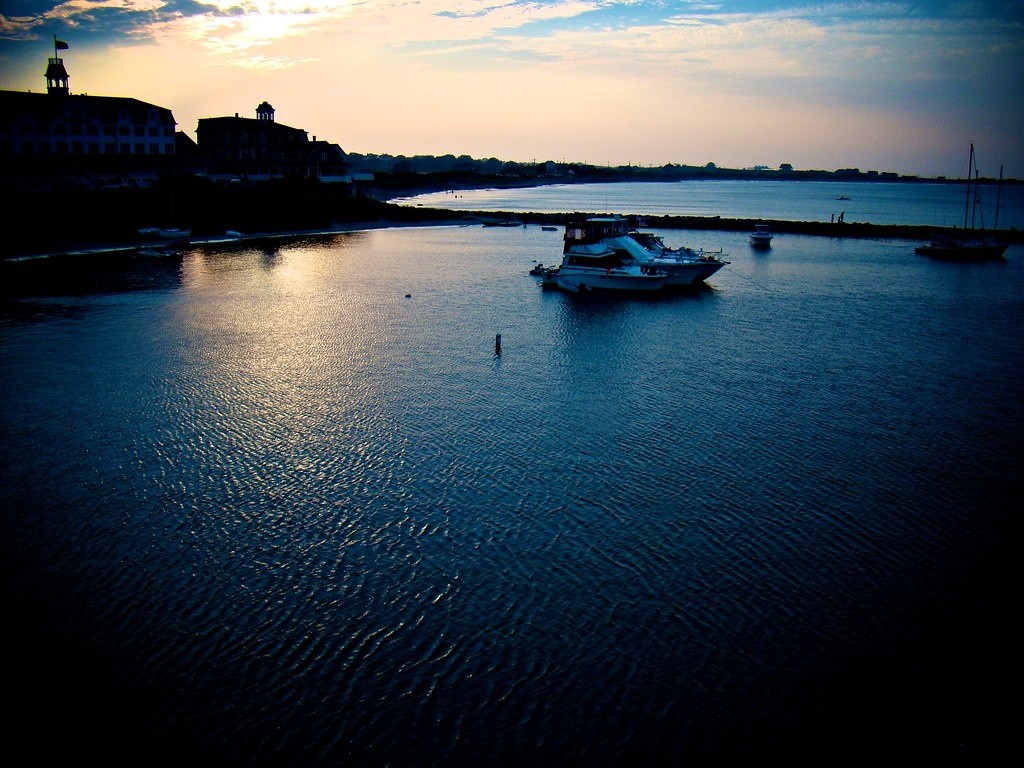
56 41 68 49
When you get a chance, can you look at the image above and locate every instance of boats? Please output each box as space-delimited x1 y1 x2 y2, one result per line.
481 220 521 226
542 226 559 231
529 193 731 295
749 225 772 250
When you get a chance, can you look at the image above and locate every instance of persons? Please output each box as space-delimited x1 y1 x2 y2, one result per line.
838 216 840 224
840 211 844 223
832 214 834 223
605 268 613 278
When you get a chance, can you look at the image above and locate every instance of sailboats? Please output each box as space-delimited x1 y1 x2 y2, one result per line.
913 143 1010 262
